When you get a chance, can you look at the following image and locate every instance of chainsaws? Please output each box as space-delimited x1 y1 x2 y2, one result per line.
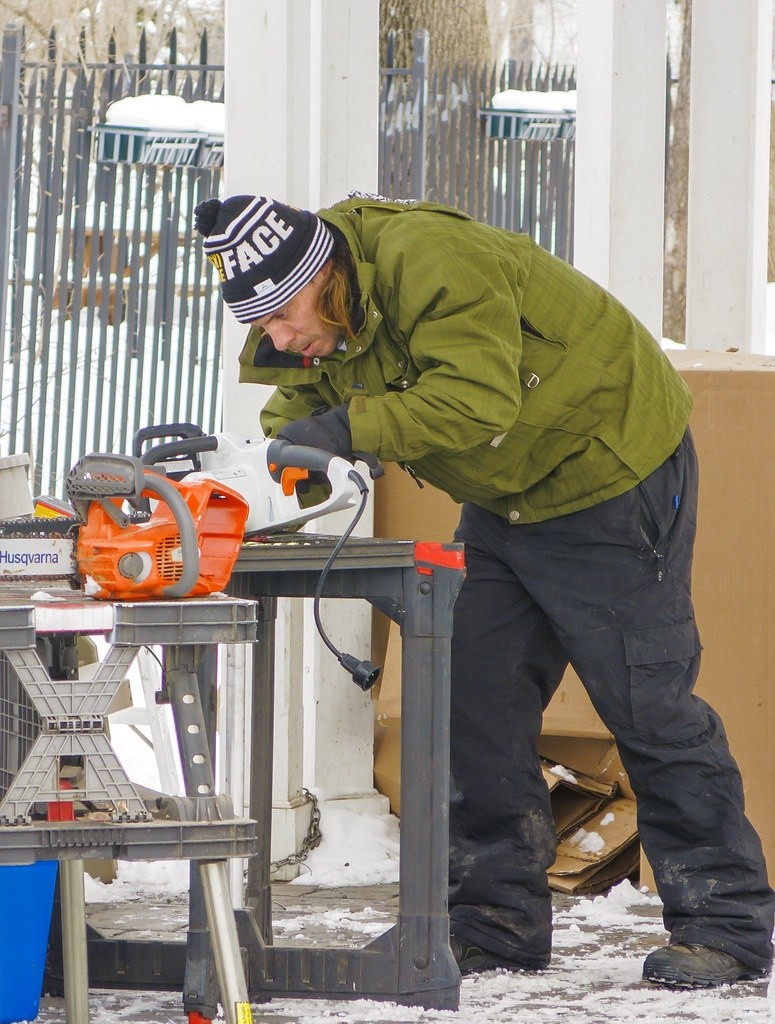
0 451 249 601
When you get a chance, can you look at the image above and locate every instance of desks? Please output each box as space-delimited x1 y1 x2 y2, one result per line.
42 537 468 1013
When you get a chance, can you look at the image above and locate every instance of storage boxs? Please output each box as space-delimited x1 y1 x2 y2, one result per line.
0 451 35 519
34 495 75 519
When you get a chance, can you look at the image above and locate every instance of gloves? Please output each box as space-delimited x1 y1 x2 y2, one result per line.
276 401 353 494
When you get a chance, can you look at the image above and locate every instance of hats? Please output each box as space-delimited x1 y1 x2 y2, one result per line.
194 194 334 325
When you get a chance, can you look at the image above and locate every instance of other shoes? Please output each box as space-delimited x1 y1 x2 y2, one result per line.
449 931 552 976
641 942 771 988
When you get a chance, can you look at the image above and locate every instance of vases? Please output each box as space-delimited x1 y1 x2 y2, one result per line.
0 859 58 1024
97 122 201 171
553 109 576 141
482 107 568 144
194 132 225 167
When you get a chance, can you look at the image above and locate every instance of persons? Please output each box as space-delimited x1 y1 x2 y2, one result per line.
194 195 775 989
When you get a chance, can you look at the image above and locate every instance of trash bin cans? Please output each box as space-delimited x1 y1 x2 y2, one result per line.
0 858 59 1023
